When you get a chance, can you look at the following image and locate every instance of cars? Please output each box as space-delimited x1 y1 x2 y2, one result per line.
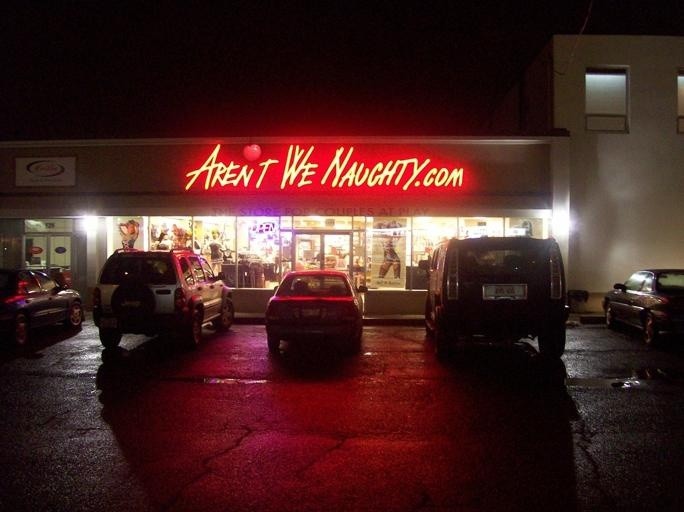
601 265 684 344
0 267 90 349
264 268 370 356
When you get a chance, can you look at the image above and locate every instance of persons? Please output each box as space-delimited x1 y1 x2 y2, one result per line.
151 231 168 251
117 220 140 253
208 235 225 277
375 218 405 281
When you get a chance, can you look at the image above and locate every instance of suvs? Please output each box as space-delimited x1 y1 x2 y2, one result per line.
91 248 234 350
418 234 572 359
